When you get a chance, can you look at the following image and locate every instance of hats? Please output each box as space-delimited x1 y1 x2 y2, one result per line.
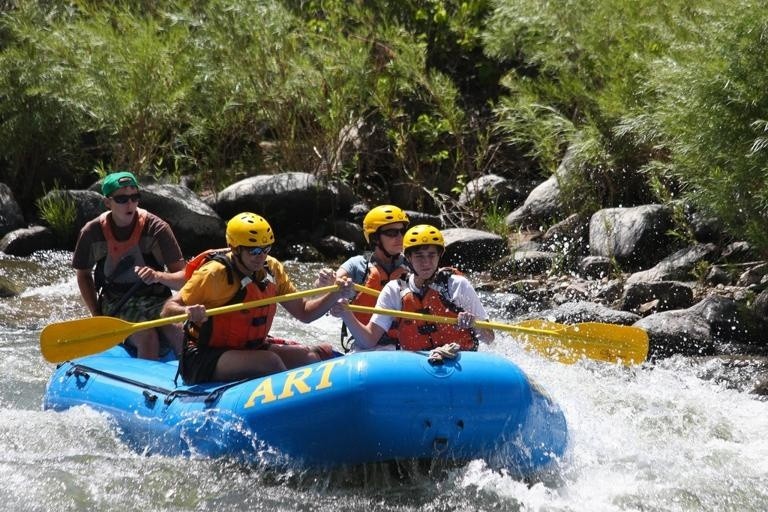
99 172 137 209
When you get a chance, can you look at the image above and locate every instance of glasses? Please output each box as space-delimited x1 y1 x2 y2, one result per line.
381 228 406 237
245 246 272 255
109 193 139 203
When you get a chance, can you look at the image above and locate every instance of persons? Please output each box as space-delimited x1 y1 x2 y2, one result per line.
330 223 496 352
315 204 412 352
160 211 357 387
70 170 188 361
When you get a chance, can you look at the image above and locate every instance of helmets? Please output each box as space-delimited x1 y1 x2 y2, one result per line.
363 205 445 258
226 212 275 247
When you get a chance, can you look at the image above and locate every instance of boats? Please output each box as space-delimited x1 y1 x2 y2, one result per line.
41 333 569 480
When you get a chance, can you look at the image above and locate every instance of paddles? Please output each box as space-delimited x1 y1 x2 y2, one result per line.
343 303 649 367
341 275 580 365
41 284 339 363
